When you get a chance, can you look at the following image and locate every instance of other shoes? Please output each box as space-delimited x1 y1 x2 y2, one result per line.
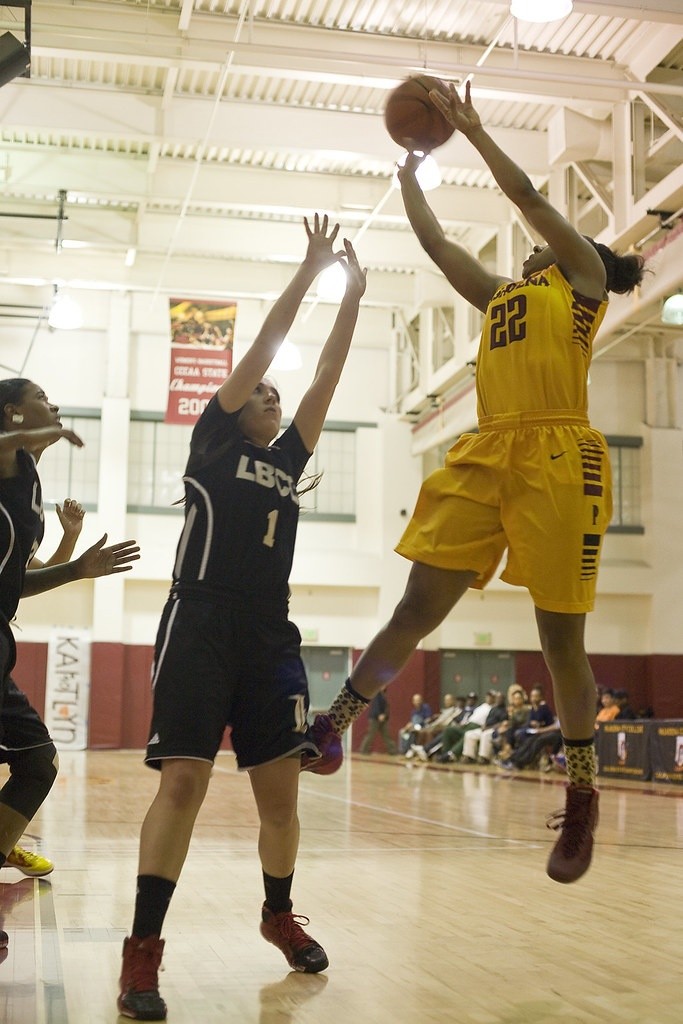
551 754 567 772
0 930 9 949
0 947 8 965
544 765 552 772
459 756 490 765
436 753 453 763
494 758 519 770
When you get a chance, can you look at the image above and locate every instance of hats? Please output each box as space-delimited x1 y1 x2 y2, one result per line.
467 692 478 701
457 697 466 702
486 689 497 697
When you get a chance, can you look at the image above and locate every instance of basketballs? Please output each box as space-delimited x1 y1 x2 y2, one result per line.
381 73 459 154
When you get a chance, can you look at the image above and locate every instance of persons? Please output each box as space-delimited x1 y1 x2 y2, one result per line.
299 71 648 884
0 376 143 956
362 686 636 770
113 207 373 1018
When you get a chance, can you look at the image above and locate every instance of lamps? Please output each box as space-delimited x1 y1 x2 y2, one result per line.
47 294 83 329
661 294 683 325
393 151 442 192
509 0 573 23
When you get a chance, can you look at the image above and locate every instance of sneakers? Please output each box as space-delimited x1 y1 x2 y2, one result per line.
260 971 328 1008
118 937 167 1021
546 783 599 883
0 878 52 918
300 714 344 775
1 845 54 877
405 744 428 762
260 905 329 974
